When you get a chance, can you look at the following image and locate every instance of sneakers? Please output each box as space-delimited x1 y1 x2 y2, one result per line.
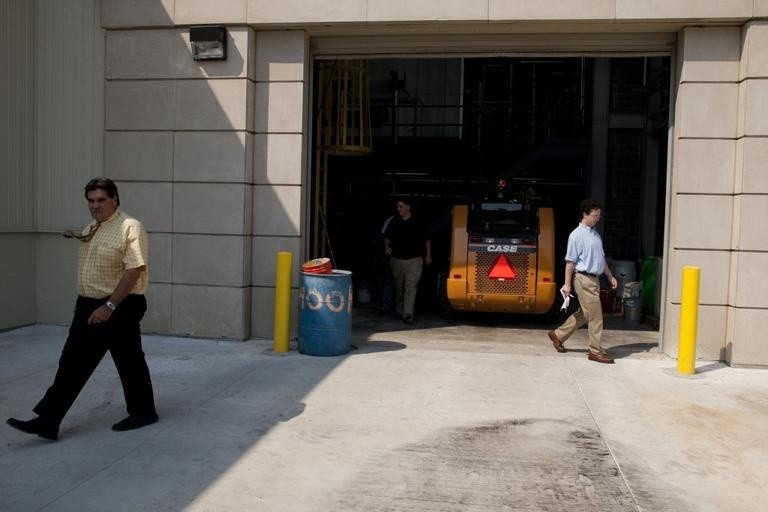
396 312 414 324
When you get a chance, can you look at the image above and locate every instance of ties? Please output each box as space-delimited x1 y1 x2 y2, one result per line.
62 222 101 242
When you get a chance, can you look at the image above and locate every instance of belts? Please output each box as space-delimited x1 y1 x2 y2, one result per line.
578 271 596 278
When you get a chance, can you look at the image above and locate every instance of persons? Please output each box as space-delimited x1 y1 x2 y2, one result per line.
5 176 161 441
381 199 433 325
547 199 619 363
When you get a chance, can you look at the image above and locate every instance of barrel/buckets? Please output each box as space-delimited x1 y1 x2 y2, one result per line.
621 299 644 325
302 257 333 274
601 288 616 314
613 258 636 296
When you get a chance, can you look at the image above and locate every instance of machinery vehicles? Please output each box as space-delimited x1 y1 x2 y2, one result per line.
446 176 559 322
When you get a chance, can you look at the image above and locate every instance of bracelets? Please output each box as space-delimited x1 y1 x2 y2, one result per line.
106 299 115 314
606 271 612 277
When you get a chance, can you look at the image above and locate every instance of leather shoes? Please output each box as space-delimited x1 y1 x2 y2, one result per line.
6 417 60 440
548 330 564 352
589 350 614 363
112 413 159 431
